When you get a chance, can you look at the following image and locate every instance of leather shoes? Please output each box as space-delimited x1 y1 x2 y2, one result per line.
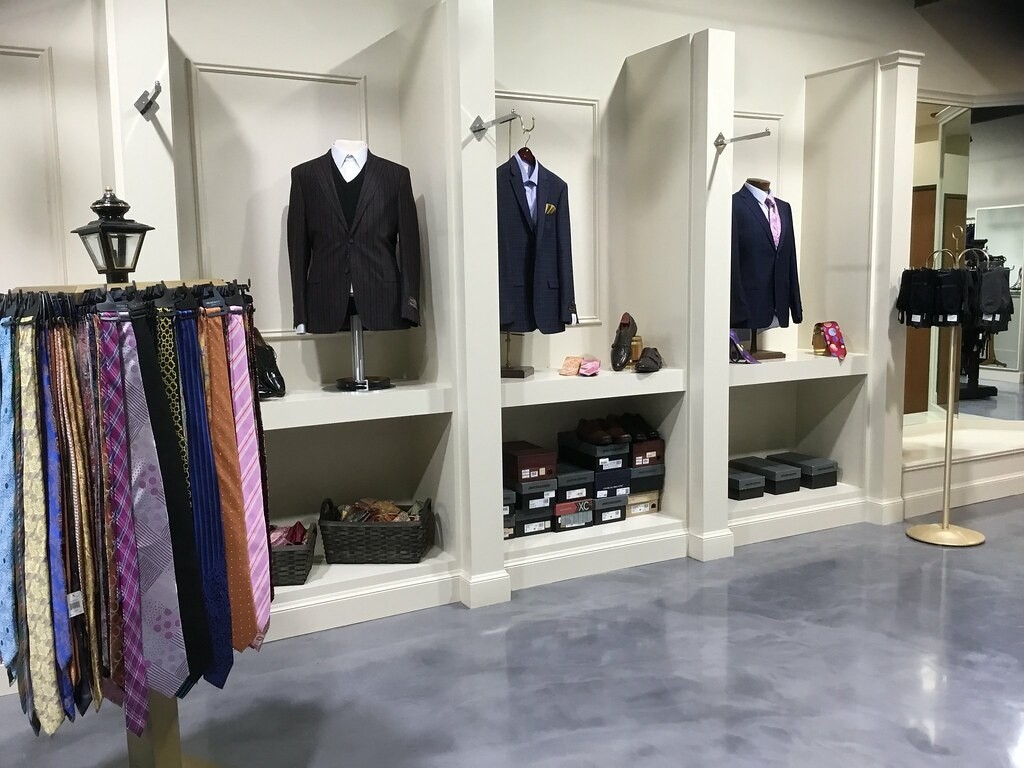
622 412 659 439
254 327 286 397
610 311 638 371
636 346 662 371
259 379 273 397
593 416 632 443
607 413 647 441
577 418 612 444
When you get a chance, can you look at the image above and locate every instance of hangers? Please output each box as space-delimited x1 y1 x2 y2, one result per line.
518 130 536 167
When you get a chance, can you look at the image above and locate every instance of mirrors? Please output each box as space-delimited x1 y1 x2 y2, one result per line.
974 205 1023 371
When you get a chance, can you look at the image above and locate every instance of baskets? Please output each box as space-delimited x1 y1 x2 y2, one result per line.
271 522 318 585
318 497 433 563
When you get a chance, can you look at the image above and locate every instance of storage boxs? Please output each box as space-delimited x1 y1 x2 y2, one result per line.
318 498 433 564
270 523 317 585
730 456 801 495
728 464 764 501
768 452 838 489
502 432 664 542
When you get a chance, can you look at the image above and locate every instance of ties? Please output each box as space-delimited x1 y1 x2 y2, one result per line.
579 358 601 375
559 356 584 375
812 321 847 359
1 304 273 737
730 329 761 363
765 195 781 250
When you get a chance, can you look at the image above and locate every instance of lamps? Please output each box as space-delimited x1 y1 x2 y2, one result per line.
70 186 154 283
